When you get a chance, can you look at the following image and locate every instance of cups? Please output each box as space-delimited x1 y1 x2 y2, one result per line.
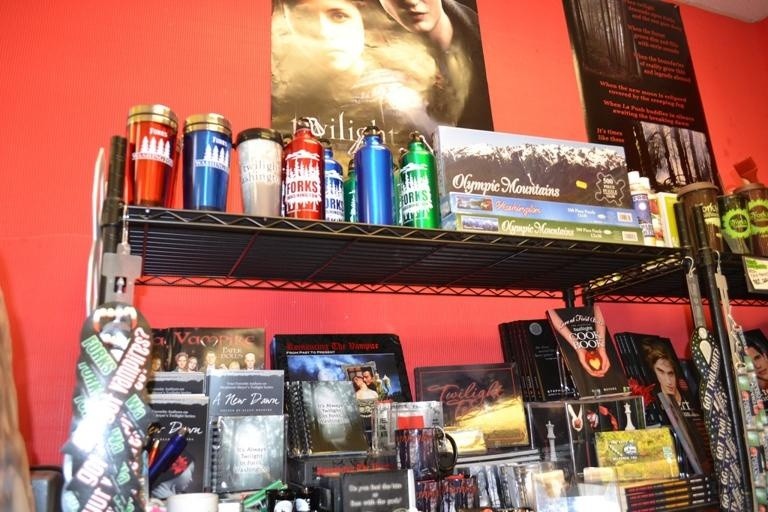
468 461 554 510
390 400 442 450
676 180 767 257
393 426 457 482
165 493 218 512
121 103 282 234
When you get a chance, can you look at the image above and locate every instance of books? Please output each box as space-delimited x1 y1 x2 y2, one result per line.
737 326 768 414
140 302 723 512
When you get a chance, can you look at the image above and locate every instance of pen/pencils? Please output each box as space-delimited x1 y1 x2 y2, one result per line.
239 478 289 509
148 426 189 479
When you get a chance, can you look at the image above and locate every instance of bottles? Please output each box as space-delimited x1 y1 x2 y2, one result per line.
279 117 440 228
626 171 660 247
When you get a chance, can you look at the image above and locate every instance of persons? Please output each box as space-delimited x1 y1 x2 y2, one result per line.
380 0 493 132
743 337 767 392
283 0 369 76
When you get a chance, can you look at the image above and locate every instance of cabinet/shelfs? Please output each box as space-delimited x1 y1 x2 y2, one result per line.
96 133 767 512
